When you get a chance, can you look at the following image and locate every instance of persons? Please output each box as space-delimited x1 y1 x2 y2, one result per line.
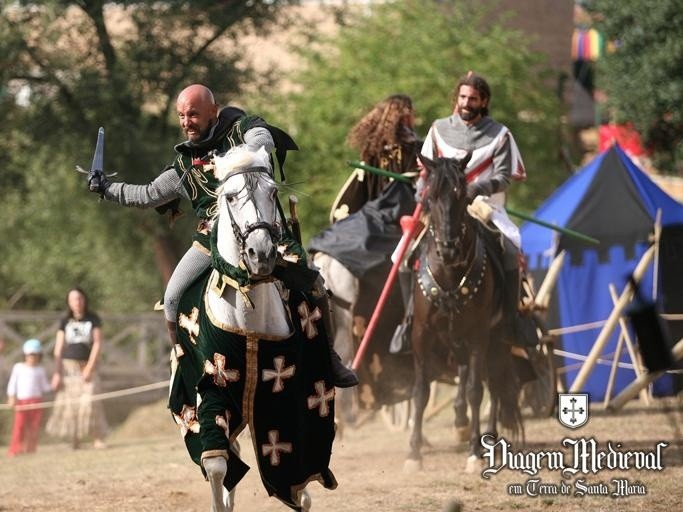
347 95 425 202
45 287 112 450
390 74 527 319
5 339 52 459
88 85 359 388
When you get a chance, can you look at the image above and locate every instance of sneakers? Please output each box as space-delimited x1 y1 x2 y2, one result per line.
330 348 359 387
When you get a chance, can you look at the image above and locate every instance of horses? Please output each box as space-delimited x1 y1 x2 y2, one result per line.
173 143 339 512
402 146 528 480
310 245 398 431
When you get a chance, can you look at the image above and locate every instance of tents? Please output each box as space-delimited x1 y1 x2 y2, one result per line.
518 135 683 403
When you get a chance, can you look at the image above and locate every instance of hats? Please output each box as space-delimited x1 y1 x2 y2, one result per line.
24 339 41 354
